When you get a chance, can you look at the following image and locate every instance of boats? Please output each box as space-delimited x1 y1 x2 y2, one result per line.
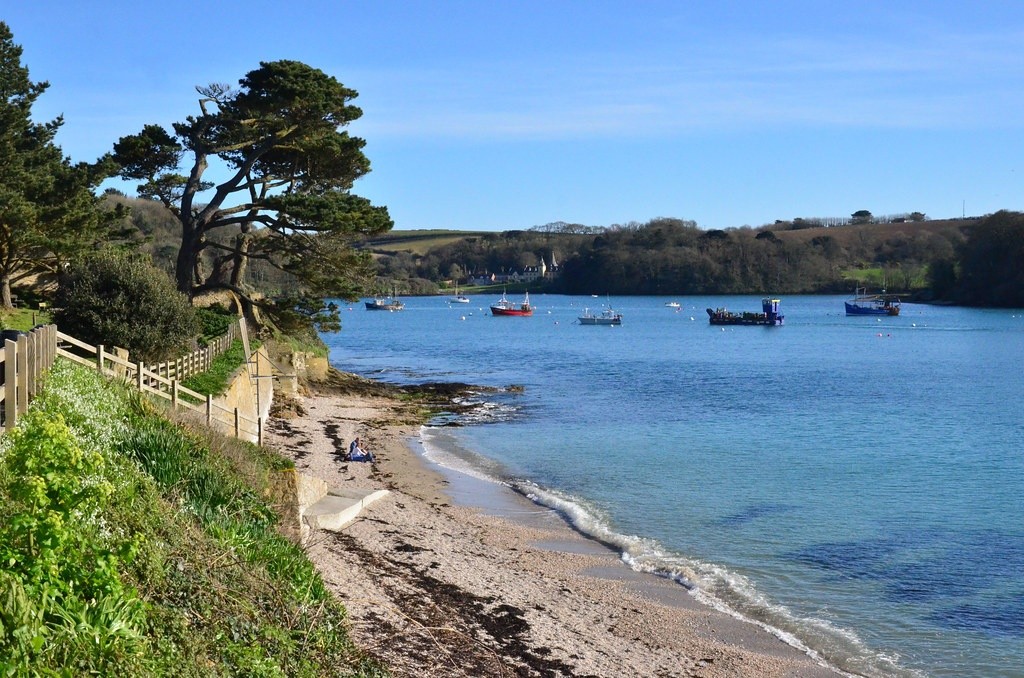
364 284 406 311
490 285 533 318
577 290 624 325
665 301 680 308
843 280 902 317
448 280 470 304
705 294 785 328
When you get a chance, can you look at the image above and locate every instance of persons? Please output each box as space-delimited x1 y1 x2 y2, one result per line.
349 437 373 461
